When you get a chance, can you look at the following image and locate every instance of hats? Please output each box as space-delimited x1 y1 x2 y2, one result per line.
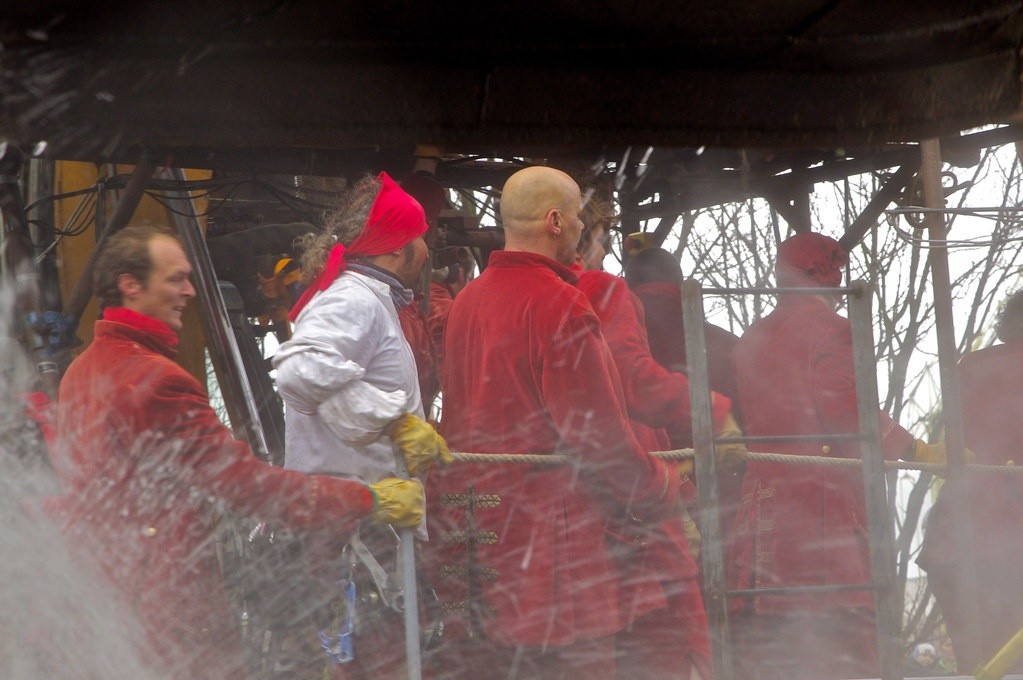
776 228 845 287
912 643 937 657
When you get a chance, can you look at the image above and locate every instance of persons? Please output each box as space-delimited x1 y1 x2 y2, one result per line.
0 141 64 443
52 225 424 680
271 166 1023 680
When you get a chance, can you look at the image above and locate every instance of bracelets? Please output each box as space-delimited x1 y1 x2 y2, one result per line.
36 359 59 375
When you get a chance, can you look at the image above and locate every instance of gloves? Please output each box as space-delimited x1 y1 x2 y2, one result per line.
680 510 702 558
906 440 974 477
369 476 424 531
388 412 458 474
718 412 748 474
